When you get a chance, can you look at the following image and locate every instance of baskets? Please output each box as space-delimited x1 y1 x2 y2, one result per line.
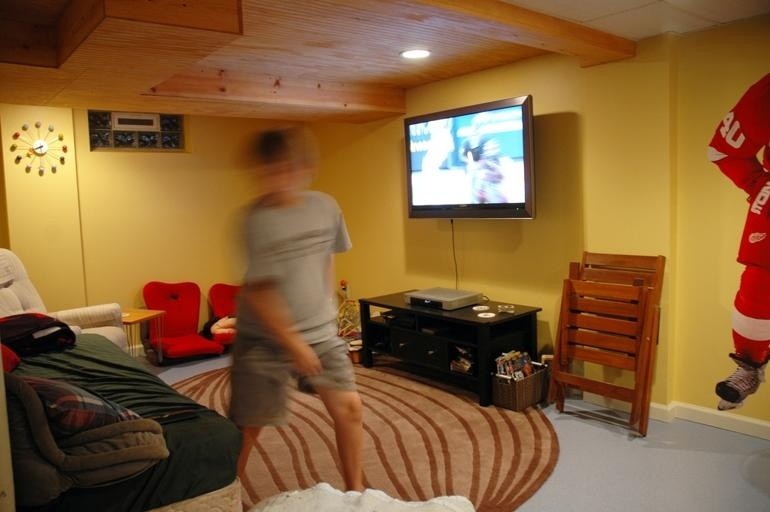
490 361 548 412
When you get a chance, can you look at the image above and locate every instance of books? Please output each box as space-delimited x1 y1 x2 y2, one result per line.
496 351 531 381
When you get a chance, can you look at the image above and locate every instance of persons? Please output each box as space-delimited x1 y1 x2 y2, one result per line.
706 74 770 402
453 125 504 204
229 131 364 492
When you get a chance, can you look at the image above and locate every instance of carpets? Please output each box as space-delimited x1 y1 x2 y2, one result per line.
165 347 560 512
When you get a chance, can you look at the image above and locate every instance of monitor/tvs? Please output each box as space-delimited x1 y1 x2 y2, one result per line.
404 95 535 221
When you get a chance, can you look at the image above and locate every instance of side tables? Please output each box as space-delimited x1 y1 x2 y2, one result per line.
120 307 163 357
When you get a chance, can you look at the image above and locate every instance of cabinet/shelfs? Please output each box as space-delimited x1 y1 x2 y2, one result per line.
357 290 543 408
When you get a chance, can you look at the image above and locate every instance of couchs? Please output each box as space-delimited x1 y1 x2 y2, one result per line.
0 250 127 352
0 332 242 512
208 283 243 346
143 281 223 362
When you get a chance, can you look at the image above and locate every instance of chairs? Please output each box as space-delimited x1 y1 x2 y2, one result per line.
548 250 666 442
